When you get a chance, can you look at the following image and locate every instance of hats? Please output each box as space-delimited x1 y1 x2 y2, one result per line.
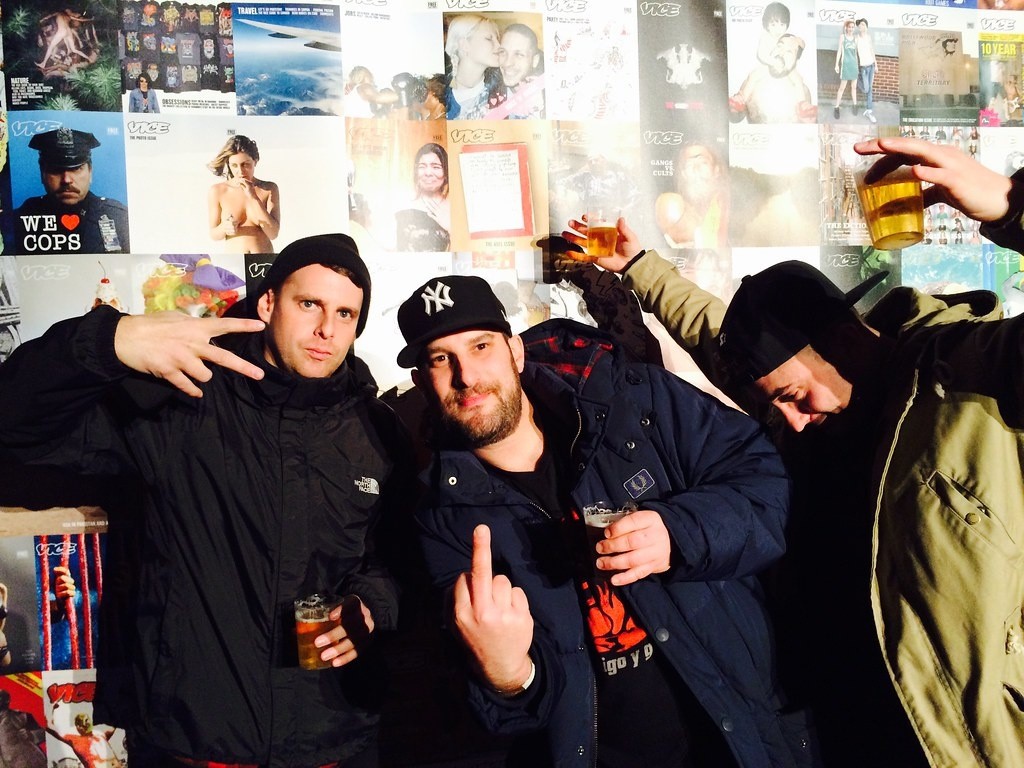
718 260 890 385
258 233 372 339
28 128 101 170
397 275 513 369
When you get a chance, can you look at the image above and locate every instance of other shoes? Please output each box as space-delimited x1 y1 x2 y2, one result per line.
863 109 877 123
834 107 840 119
853 104 858 116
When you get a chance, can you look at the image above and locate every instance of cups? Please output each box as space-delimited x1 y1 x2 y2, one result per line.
848 146 925 251
587 210 623 257
582 499 641 577
294 592 343 670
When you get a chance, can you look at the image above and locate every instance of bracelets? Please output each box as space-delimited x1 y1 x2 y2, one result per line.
492 658 535 697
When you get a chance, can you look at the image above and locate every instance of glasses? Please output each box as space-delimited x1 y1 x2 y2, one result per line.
139 80 148 83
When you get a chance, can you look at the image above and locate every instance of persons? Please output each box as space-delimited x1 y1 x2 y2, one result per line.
49 563 75 622
42 709 123 768
20 1 1024 247
0 585 10 667
0 137 1024 768
